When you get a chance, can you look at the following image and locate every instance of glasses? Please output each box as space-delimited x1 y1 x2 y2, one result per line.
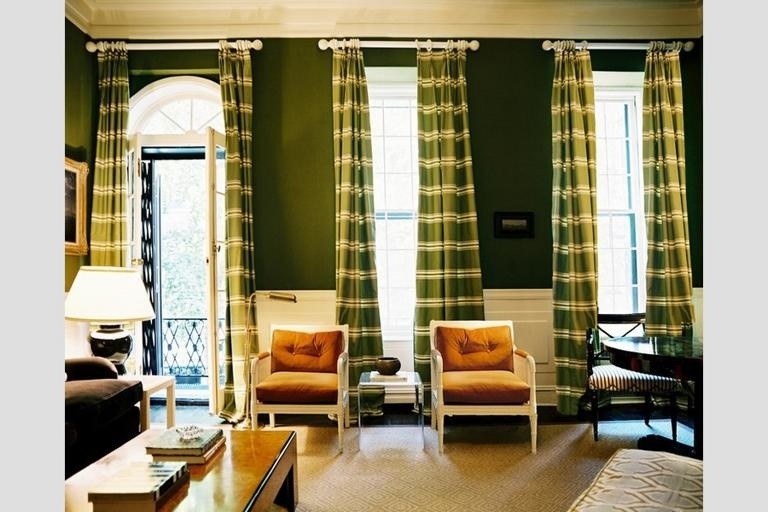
231 290 298 425
64 265 157 375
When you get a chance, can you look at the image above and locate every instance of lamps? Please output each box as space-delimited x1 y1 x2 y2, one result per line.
587 313 680 448
249 323 351 455
429 318 539 456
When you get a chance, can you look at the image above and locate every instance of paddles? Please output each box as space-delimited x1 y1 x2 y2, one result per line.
564 444 704 512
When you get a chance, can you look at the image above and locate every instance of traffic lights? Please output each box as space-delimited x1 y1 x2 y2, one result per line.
436 325 514 372
270 328 343 375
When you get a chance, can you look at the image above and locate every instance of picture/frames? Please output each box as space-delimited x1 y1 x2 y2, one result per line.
602 335 703 460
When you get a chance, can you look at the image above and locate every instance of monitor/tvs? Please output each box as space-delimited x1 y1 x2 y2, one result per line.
87 426 226 512
